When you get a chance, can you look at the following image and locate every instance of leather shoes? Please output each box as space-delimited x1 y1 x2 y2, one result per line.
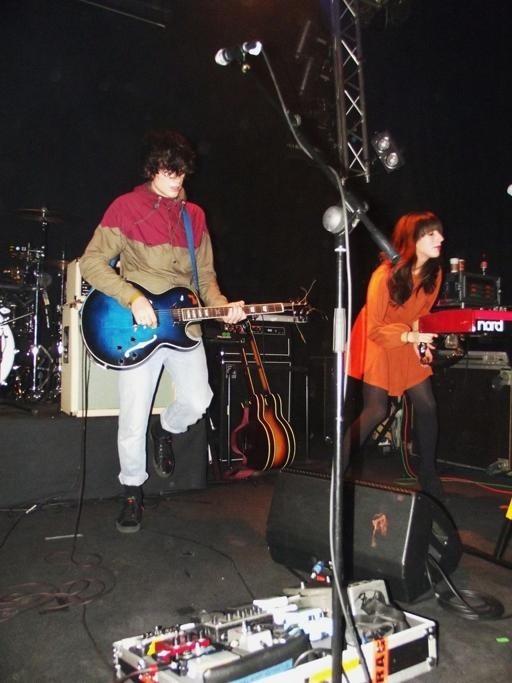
116 486 144 535
151 419 175 478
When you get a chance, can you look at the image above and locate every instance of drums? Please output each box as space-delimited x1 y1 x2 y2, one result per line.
0 300 35 391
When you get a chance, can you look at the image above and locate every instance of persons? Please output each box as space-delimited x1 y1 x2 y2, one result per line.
341 208 449 540
79 129 249 535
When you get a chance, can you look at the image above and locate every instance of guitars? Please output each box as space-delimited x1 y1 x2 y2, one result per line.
78 280 312 370
231 320 297 473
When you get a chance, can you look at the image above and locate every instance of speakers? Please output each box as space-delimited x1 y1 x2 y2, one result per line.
211 358 294 465
266 467 463 604
410 363 512 475
60 302 175 418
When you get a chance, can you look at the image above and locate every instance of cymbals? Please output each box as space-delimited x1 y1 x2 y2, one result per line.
11 208 79 223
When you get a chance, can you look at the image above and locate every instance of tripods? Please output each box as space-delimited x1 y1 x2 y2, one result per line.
12 221 58 401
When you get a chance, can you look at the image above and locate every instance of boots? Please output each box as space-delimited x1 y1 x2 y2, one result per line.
421 479 451 507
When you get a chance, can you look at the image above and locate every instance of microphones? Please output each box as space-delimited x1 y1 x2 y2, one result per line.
215 41 262 66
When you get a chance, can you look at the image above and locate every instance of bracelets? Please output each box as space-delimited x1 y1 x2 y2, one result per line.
129 290 143 306
406 330 410 343
